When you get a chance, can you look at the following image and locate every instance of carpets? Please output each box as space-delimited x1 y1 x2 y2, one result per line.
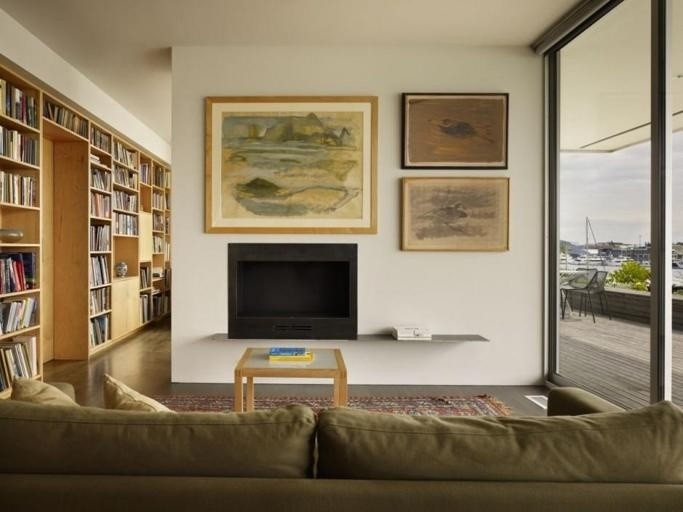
151 392 512 418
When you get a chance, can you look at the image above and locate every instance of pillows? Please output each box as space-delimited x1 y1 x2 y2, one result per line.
102 373 177 415
12 377 82 409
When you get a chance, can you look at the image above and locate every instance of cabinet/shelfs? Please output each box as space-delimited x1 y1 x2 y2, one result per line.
0 65 44 400
53 120 172 361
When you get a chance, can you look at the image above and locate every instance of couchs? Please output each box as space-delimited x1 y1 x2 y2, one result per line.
0 387 683 512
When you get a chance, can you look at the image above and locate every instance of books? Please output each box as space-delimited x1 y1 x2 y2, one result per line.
268 347 305 355
269 352 313 361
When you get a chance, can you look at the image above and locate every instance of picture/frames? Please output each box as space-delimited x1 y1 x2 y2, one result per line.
204 95 376 235
401 92 510 169
398 176 509 252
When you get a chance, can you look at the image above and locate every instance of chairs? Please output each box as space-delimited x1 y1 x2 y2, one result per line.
565 270 610 322
560 268 604 316
